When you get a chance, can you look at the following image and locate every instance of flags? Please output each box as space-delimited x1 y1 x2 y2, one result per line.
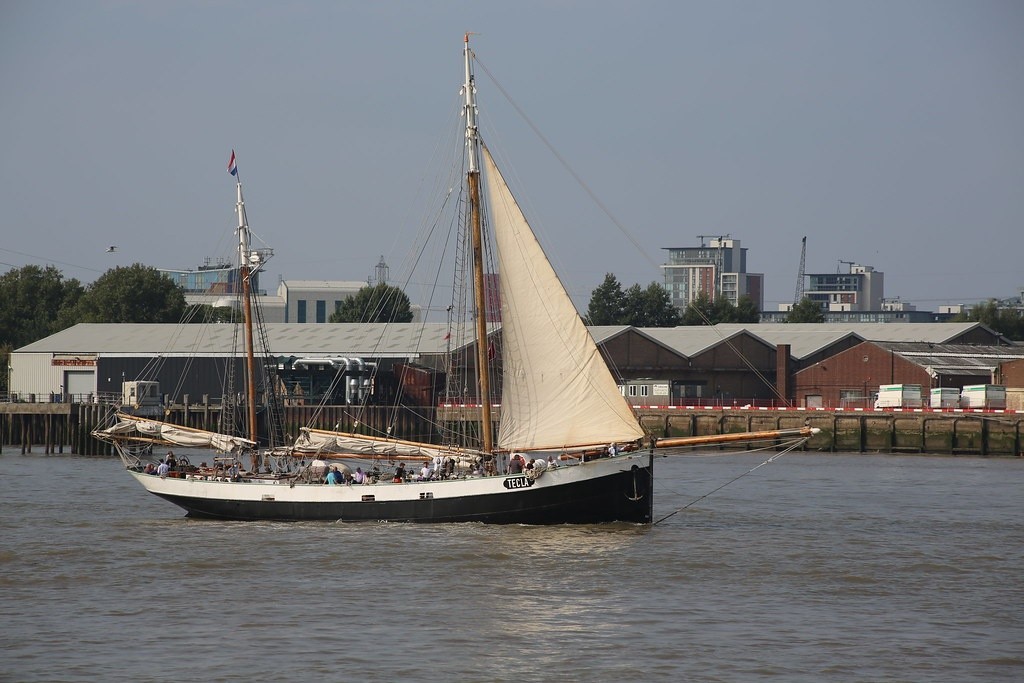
226 148 238 176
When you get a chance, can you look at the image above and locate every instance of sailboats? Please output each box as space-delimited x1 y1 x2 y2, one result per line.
88 29 657 526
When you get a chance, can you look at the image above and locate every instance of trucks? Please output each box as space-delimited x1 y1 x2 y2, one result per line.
923 387 961 409
873 383 923 412
959 383 1007 409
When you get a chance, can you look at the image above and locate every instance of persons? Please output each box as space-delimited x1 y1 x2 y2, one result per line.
143 441 626 485
